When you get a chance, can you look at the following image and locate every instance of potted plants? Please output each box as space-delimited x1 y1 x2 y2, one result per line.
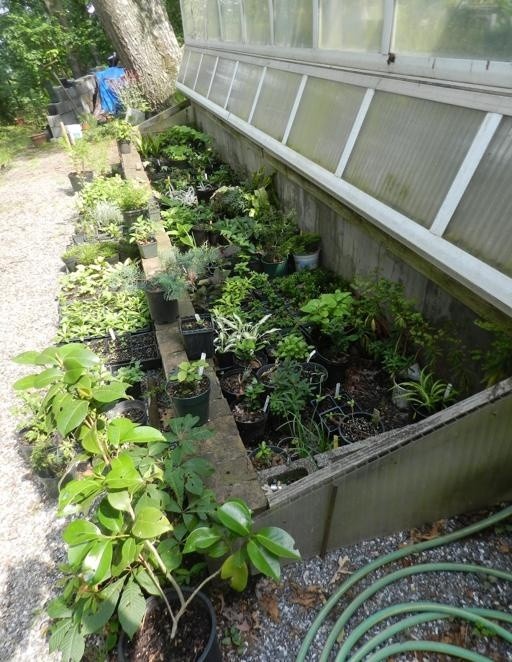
12 94 486 661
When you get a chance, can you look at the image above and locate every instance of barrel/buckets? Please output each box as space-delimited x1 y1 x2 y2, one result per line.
64 123 82 145
